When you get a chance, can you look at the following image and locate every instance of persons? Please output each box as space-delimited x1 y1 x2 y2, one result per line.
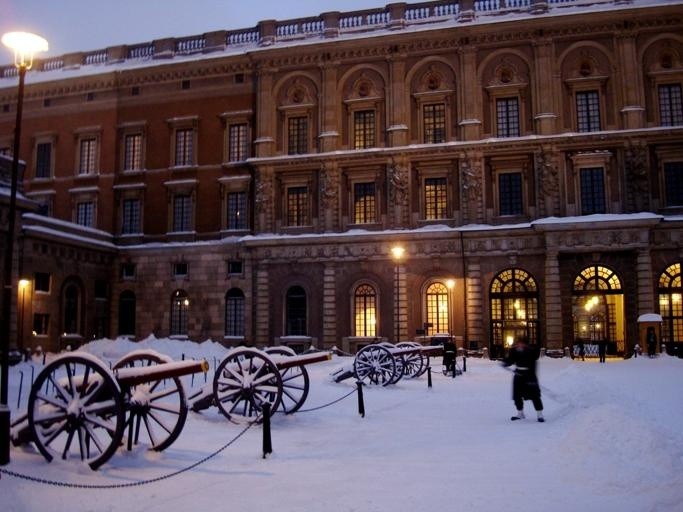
646 327 657 359
598 335 607 363
501 334 545 422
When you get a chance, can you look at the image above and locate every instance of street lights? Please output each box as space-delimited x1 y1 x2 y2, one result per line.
391 243 405 342
447 280 455 339
1 30 49 467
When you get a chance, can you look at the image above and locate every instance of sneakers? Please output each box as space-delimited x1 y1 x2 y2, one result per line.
511 417 520 421
537 417 544 423
